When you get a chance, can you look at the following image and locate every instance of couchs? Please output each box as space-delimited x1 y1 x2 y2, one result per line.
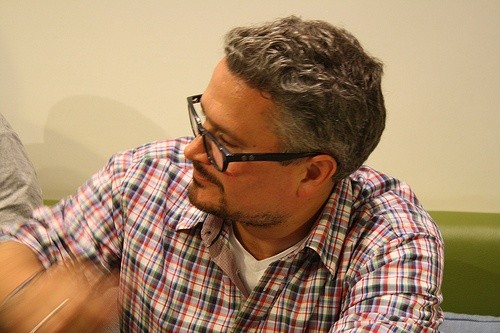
44 198 497 333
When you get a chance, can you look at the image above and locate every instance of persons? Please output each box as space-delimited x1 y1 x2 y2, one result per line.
2 13 446 333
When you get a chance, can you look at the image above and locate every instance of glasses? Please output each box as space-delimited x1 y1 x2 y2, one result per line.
186 95 331 172
